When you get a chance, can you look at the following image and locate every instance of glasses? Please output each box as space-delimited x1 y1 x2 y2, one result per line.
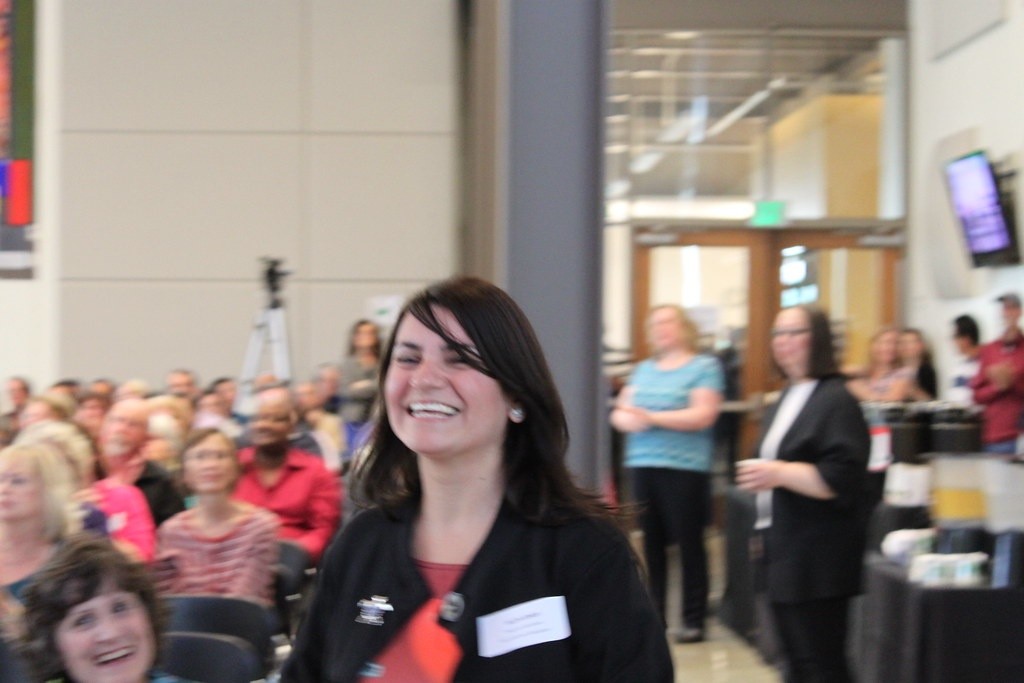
769 327 812 339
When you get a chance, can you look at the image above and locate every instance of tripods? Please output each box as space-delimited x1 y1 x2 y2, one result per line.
232 289 295 419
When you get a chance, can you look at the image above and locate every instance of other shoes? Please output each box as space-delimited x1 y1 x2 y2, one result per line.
678 625 704 642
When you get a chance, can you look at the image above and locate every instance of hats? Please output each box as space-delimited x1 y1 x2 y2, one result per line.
998 294 1021 309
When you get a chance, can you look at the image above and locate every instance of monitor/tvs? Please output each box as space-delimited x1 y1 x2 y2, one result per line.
945 148 1021 268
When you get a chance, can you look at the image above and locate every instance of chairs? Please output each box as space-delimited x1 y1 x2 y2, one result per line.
0 539 312 683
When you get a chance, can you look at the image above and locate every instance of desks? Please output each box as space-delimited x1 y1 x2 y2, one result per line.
717 483 1024 682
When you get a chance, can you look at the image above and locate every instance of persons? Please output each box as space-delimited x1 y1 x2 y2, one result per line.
273 273 676 683
607 303 725 644
734 302 872 683
845 293 1024 455
1 318 385 683
